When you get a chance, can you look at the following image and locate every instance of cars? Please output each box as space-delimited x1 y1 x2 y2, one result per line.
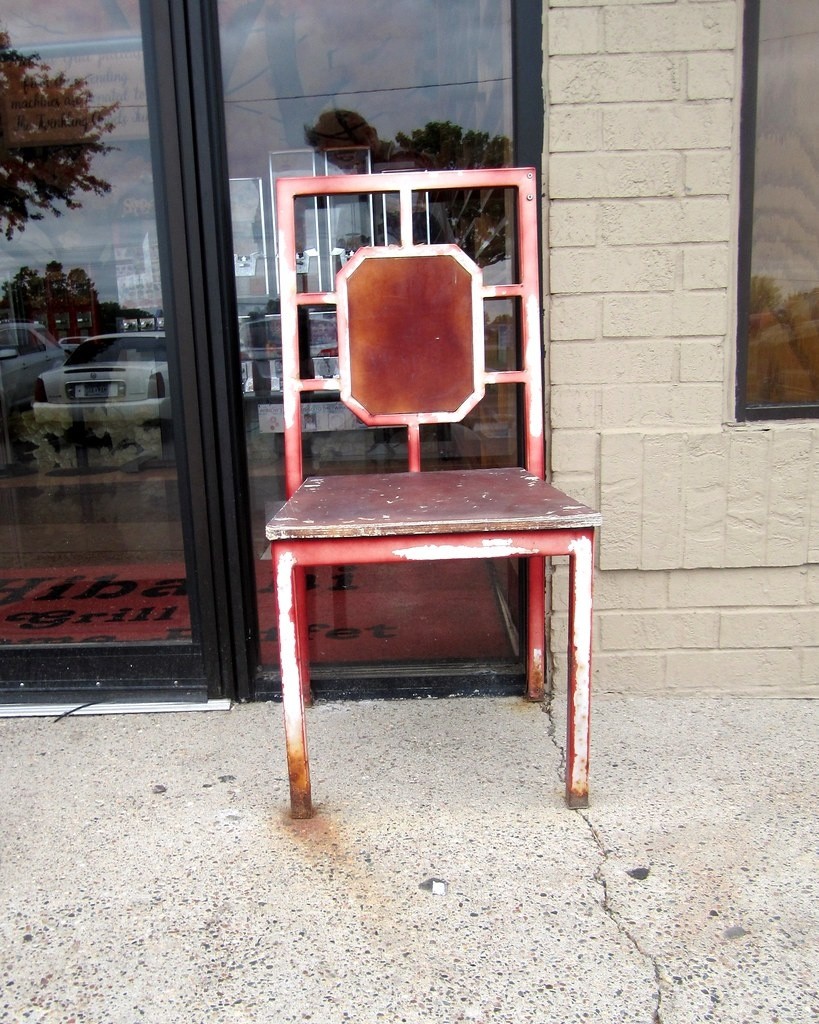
57 336 91 356
29 331 173 445
237 300 521 422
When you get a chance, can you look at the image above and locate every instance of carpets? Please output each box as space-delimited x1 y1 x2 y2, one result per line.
0 561 512 668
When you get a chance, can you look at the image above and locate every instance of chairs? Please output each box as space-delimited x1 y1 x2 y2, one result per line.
267 166 604 819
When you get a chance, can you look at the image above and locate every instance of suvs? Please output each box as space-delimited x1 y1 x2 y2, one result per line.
0 320 71 418
